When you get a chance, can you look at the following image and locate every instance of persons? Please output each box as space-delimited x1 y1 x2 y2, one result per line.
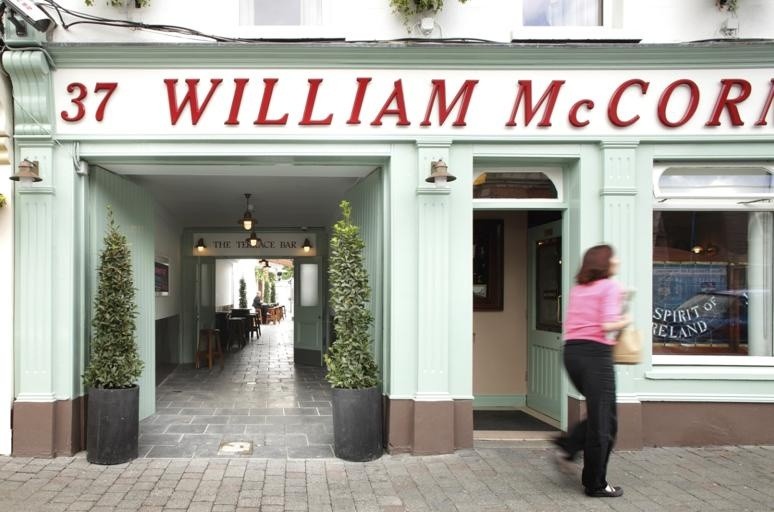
552 244 635 496
252 291 272 317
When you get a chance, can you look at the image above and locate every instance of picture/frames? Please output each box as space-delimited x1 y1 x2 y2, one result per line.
155 256 170 297
474 218 504 312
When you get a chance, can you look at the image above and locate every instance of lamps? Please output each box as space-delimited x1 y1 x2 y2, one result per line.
193 236 207 253
424 159 457 190
245 230 261 247
237 194 258 232
8 157 42 192
300 237 313 253
258 258 271 271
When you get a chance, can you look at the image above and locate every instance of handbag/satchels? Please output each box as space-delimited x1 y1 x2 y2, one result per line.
610 324 644 367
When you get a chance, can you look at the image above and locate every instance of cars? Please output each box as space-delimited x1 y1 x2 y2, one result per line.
665 288 748 346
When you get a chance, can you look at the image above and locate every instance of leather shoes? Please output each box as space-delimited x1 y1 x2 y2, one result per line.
585 483 623 499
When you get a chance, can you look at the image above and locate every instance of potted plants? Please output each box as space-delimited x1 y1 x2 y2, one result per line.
81 205 145 466
322 200 384 462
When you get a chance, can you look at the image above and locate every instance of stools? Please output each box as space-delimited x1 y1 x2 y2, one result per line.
194 328 225 369
217 302 286 352
